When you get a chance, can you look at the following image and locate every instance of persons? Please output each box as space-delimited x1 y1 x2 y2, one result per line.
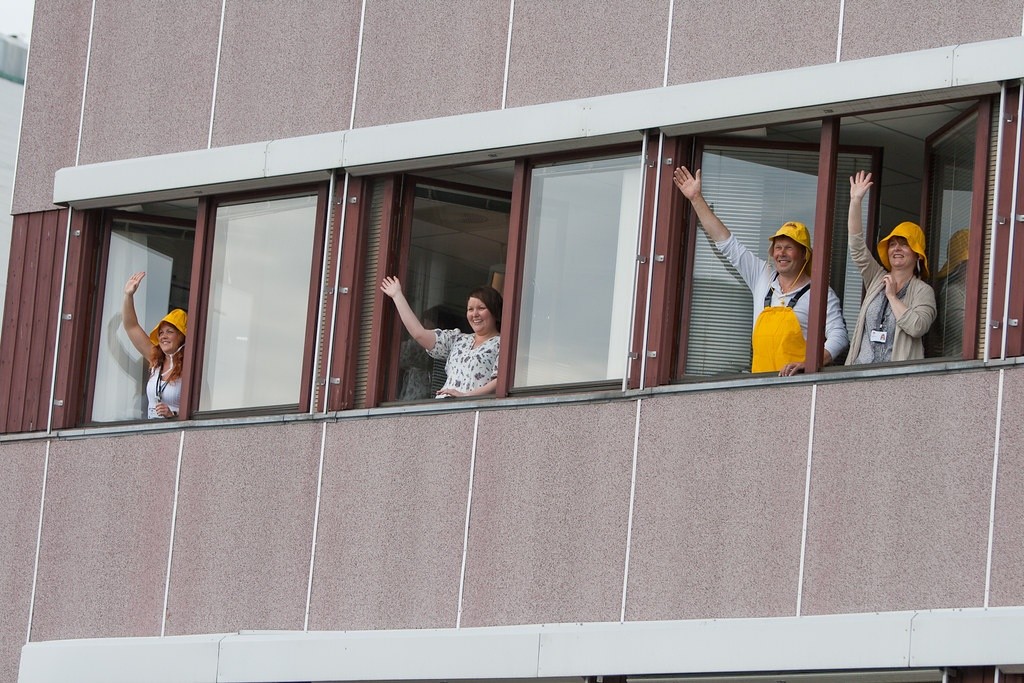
673 166 849 378
925 228 969 358
846 170 935 367
110 313 156 418
381 276 503 399
124 271 187 419
881 334 887 341
400 304 454 400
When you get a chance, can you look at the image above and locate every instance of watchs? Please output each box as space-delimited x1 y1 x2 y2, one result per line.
172 411 178 417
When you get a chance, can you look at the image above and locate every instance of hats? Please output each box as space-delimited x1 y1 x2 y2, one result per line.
938 229 970 278
768 221 814 268
877 221 930 277
149 309 187 346
